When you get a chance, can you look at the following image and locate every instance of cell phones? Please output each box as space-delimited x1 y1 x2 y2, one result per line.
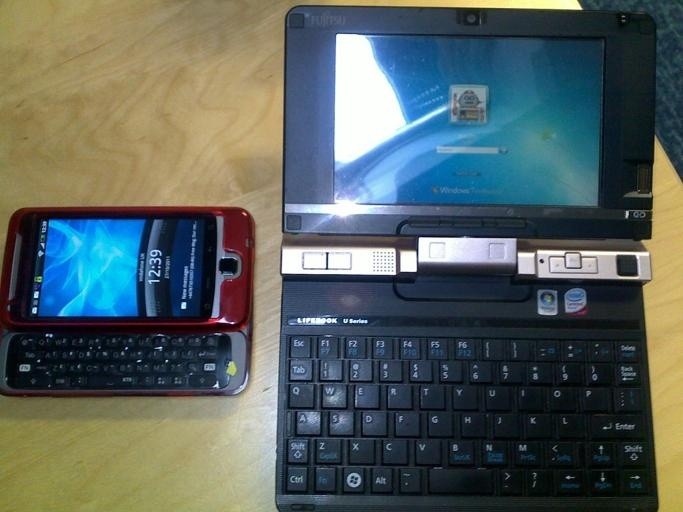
0 205 256 398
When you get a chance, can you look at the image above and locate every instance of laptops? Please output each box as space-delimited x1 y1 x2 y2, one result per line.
273 3 661 512
